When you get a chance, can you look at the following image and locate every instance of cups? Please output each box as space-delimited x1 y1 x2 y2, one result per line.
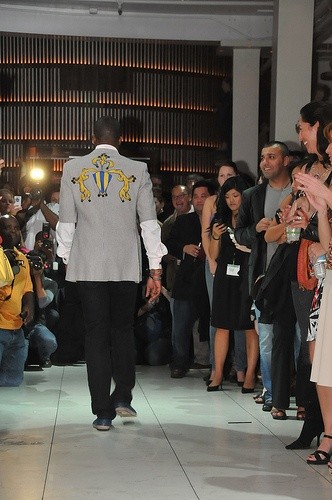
283 215 301 242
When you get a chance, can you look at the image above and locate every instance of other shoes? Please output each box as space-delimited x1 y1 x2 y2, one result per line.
263 403 271 411
204 374 210 381
206 379 212 386
230 374 237 382
236 372 243 387
255 397 264 403
253 392 263 399
171 367 185 378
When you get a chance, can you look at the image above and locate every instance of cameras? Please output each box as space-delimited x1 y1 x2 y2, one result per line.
31 187 42 200
40 221 50 249
30 259 43 271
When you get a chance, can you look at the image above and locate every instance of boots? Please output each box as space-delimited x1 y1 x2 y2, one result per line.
286 365 324 449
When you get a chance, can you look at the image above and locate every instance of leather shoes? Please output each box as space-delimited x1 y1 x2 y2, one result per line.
92 418 111 431
113 401 136 417
40 360 51 367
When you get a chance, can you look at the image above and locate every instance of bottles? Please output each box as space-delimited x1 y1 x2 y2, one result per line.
312 263 327 281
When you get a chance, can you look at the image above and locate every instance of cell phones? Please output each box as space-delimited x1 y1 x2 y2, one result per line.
215 219 223 230
15 196 22 206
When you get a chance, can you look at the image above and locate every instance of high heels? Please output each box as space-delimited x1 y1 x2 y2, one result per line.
242 378 256 393
207 379 222 391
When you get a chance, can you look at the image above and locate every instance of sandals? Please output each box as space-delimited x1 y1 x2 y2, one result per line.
328 460 332 469
271 406 288 420
307 435 332 465
297 406 306 420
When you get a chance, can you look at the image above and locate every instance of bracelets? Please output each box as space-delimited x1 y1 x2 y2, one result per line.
212 234 220 240
149 269 162 280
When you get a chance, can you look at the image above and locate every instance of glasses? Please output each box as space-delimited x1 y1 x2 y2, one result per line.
172 194 187 199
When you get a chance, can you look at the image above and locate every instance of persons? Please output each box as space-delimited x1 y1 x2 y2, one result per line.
152 102 332 500
0 182 60 387
56 116 167 430
